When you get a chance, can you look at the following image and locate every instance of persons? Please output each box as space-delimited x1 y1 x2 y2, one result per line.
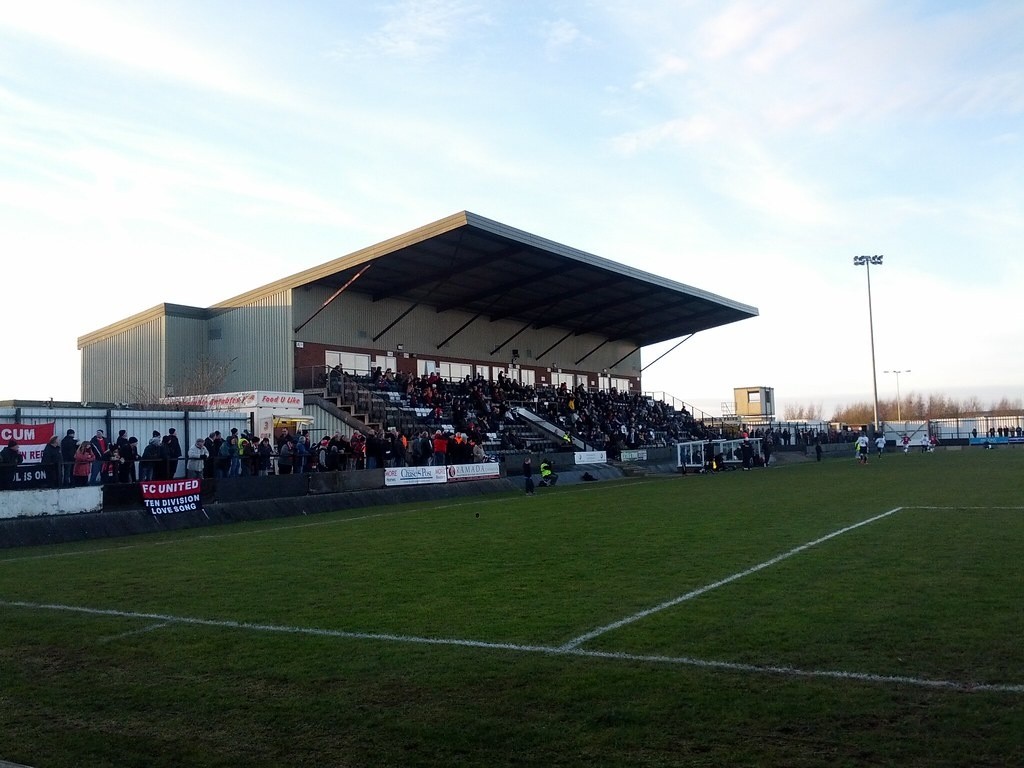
920 432 941 454
329 364 869 501
900 433 911 455
0 427 330 491
875 434 886 458
989 425 1024 438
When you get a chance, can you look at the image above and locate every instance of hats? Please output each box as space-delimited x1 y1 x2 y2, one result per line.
169 427 176 434
321 440 328 446
196 438 204 442
129 436 138 443
109 443 121 452
119 429 126 436
231 428 238 434
436 430 441 434
460 433 468 440
162 435 171 443
152 429 160 437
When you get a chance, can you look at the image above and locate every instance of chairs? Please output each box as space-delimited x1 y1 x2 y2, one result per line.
351 390 454 433
482 420 556 457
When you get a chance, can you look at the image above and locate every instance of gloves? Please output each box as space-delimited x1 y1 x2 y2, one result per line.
200 454 207 459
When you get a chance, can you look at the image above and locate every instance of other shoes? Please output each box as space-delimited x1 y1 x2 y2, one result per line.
525 493 529 495
530 492 537 495
547 482 554 487
